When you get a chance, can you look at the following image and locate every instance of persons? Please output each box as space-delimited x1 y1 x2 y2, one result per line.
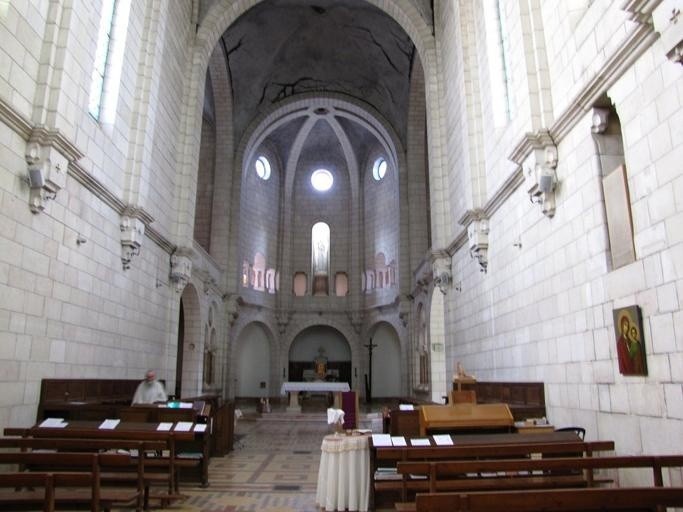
130 367 167 404
617 314 634 375
627 325 642 375
312 346 328 380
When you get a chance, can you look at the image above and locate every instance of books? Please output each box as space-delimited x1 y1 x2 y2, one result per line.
367 402 542 481
192 423 207 433
173 420 194 432
46 421 68 428
38 416 63 427
98 418 120 430
31 447 203 460
156 421 173 431
157 400 193 409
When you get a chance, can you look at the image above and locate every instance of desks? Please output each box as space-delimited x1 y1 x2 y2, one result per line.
318 429 369 511
30 378 235 490
370 380 584 444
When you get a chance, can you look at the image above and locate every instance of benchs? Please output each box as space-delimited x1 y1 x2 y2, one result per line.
0 428 194 512
